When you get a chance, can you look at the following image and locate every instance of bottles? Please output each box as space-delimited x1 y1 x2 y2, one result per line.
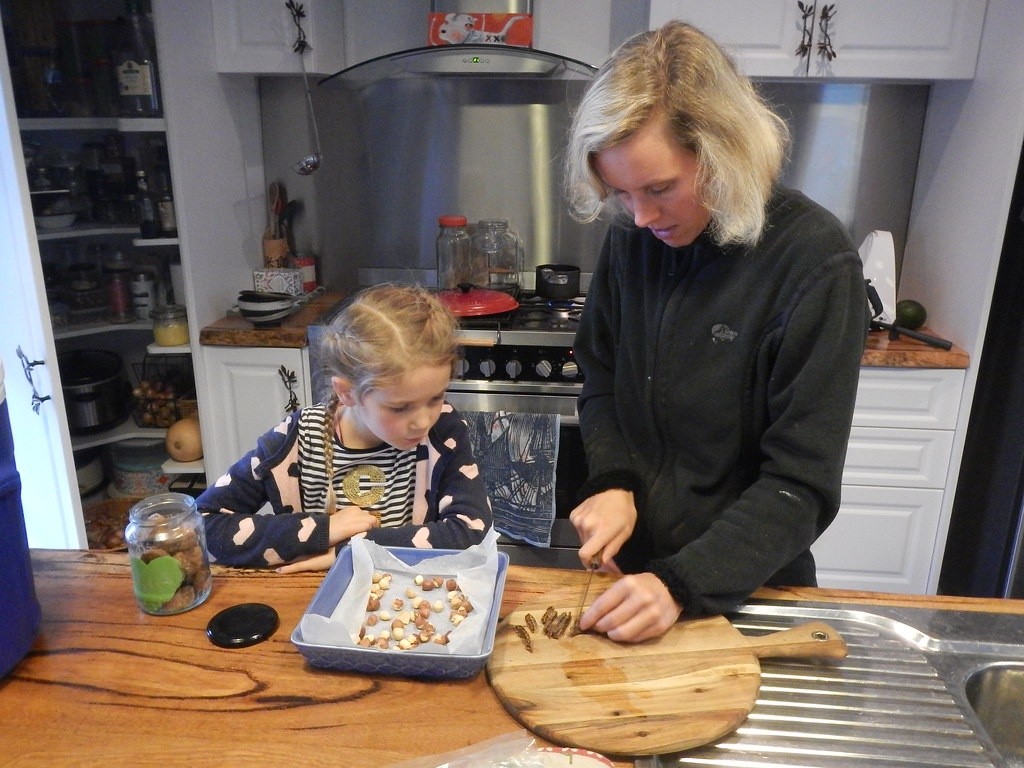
436 217 472 288
85 144 120 222
470 219 519 288
135 170 176 239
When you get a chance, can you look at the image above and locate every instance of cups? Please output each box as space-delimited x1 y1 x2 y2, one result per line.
113 3 162 118
292 256 317 292
261 239 286 268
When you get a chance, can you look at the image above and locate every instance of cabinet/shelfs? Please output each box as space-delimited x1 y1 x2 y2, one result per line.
810 368 979 596
212 0 344 76
190 346 313 490
648 0 989 85
0 0 270 549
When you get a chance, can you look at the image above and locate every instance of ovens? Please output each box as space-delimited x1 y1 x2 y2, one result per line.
443 389 595 569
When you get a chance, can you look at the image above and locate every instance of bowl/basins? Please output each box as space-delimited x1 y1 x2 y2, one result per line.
32 190 78 228
239 291 294 326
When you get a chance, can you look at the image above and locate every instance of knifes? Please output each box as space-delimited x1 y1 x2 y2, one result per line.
575 548 604 624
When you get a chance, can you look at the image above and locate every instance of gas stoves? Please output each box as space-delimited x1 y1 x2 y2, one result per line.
307 289 587 394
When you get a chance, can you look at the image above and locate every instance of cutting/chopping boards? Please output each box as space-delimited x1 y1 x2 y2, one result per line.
485 581 849 757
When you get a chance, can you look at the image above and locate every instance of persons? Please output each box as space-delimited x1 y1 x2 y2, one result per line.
566 16 870 643
196 285 493 574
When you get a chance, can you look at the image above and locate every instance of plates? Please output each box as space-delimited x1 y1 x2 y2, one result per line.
537 747 615 768
290 544 511 678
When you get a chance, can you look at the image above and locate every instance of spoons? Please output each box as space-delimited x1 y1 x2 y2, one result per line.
268 182 279 238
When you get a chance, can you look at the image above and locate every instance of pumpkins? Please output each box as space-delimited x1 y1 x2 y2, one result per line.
166 413 203 461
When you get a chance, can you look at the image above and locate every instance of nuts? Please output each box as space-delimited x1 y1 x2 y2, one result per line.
358 574 473 651
139 522 211 609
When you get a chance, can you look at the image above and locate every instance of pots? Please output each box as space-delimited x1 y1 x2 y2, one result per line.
536 264 581 299
57 349 129 435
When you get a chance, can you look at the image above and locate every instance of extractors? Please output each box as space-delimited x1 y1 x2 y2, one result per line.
315 0 598 108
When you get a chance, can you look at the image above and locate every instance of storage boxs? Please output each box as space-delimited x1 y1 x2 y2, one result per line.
254 268 304 296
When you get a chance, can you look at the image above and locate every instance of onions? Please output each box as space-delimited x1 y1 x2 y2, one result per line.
132 380 176 427
85 514 129 550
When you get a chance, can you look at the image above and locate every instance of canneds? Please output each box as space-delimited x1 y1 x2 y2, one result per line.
82 134 138 226
22 18 121 117
150 306 190 346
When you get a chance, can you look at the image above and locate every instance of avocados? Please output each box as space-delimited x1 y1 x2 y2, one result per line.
896 300 927 329
